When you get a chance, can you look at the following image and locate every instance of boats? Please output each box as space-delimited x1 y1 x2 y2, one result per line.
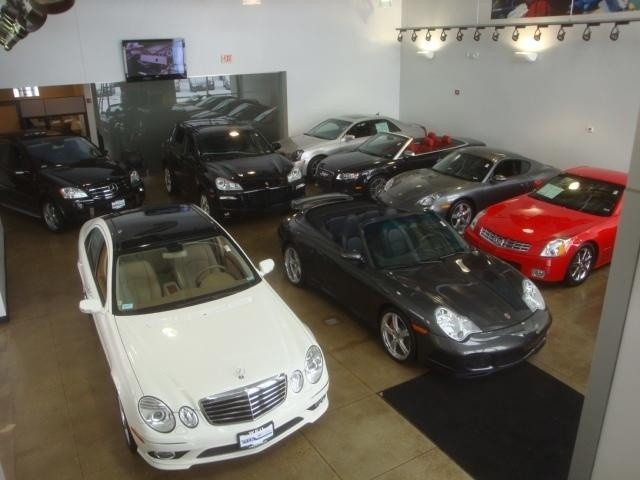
222 76 231 90
187 77 215 92
174 79 181 93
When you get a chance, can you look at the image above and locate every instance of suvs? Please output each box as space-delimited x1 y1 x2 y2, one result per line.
1 126 146 233
161 116 305 225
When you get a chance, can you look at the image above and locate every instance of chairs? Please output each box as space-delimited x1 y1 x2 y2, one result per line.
322 215 353 247
115 259 161 304
360 210 382 226
386 227 407 256
345 233 369 261
169 243 222 289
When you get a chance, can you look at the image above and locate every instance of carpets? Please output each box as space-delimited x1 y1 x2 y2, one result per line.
376 354 586 478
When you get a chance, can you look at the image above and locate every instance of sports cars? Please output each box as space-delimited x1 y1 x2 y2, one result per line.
378 146 562 236
278 191 552 383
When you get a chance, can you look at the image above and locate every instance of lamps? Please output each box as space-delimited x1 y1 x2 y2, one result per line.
394 18 640 42
0 0 75 51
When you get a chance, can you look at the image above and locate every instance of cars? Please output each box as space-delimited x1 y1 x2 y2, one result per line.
314 130 486 201
171 95 280 143
465 164 630 286
271 113 428 179
77 202 331 471
99 103 149 133
